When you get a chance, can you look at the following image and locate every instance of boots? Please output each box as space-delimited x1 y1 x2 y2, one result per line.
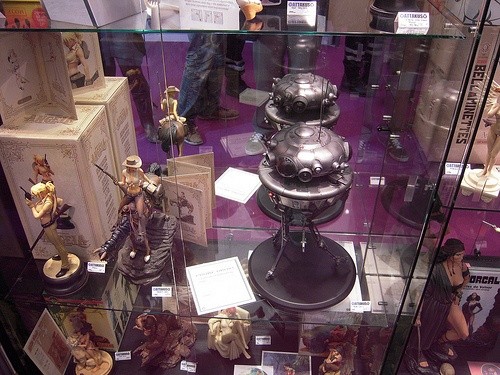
131 85 160 143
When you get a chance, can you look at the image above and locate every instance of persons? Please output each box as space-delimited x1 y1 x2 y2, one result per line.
115 155 151 263
412 239 470 367
96 30 162 143
178 33 240 146
209 307 252 359
134 310 183 358
71 327 102 373
25 181 70 277
319 341 345 375
158 85 186 125
384 244 432 328
337 37 379 95
63 31 91 89
478 94 500 179
227 34 251 99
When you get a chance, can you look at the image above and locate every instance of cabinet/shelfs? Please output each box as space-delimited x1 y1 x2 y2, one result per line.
0 0 500 375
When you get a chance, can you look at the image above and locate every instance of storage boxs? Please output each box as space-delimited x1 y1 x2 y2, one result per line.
72 76 138 198
0 104 120 263
412 0 500 80
40 261 142 352
410 62 500 167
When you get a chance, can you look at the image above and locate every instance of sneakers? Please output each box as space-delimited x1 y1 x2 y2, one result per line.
182 119 203 144
378 132 409 162
201 106 239 120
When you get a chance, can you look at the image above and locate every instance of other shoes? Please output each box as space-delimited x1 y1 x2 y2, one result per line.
225 78 250 99
